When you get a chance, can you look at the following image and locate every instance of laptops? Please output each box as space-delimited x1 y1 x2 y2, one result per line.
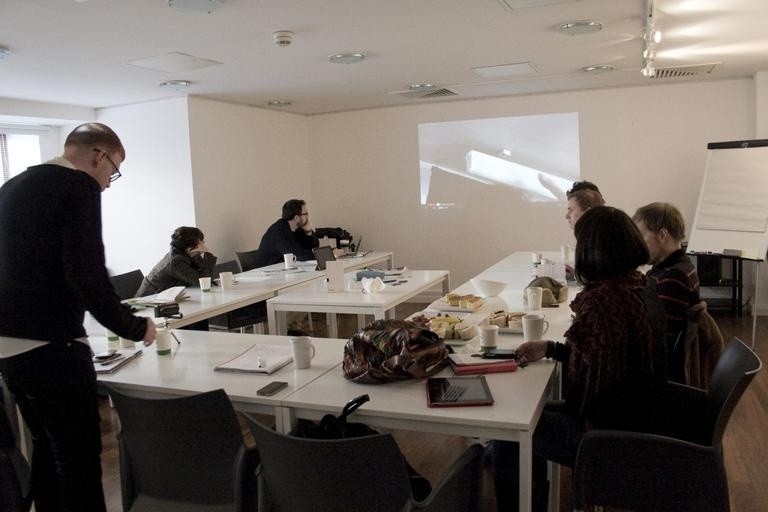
338 235 362 257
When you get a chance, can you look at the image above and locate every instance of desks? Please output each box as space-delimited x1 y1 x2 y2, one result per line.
401 271 587 343
79 330 353 435
82 287 275 335
483 251 580 281
279 344 560 510
264 268 450 337
215 248 395 335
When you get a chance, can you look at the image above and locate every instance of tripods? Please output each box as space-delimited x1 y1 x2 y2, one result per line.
158 323 180 346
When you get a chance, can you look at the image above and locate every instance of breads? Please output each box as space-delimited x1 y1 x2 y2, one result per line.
446 293 482 309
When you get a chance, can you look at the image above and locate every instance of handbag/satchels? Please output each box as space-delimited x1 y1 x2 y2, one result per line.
341 317 453 386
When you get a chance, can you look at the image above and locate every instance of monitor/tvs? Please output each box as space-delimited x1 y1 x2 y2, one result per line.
311 245 336 270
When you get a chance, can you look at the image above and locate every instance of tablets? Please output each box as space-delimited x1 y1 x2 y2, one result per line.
426 374 495 408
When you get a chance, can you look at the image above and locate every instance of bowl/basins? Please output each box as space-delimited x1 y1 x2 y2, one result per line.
470 279 507 297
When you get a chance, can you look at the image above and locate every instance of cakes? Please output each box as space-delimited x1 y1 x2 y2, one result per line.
488 310 527 329
425 312 475 340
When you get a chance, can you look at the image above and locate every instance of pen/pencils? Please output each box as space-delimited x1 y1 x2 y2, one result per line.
101 357 125 365
170 331 182 343
211 280 218 286
255 357 262 369
381 280 396 283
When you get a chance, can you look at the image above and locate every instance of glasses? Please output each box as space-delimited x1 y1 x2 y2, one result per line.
91 147 122 184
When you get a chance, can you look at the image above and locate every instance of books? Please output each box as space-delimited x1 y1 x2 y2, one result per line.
448 357 518 375
95 349 144 375
137 287 191 306
212 343 294 376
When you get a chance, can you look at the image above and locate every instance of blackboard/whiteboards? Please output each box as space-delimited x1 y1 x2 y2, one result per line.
687 140 768 262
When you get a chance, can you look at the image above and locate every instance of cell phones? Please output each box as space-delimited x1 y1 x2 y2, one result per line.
256 381 288 396
96 349 117 358
484 347 517 360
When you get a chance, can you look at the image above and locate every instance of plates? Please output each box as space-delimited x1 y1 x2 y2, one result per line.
429 298 486 313
476 318 524 334
444 331 480 346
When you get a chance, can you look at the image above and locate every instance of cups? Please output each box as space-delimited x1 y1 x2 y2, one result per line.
198 278 211 292
219 271 236 290
527 287 542 310
290 336 316 370
155 328 172 355
561 246 570 261
340 239 349 253
532 252 542 266
479 324 500 352
521 314 549 342
324 261 345 294
284 253 297 267
105 327 120 346
119 335 134 348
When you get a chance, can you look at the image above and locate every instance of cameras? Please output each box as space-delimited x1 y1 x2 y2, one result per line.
154 302 179 319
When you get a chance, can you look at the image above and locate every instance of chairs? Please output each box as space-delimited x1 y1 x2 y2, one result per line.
312 246 357 327
237 408 488 510
569 337 761 509
109 270 146 299
663 302 710 388
208 260 261 333
236 251 314 334
97 381 275 511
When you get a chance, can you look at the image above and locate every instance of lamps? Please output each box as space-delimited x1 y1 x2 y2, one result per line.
638 24 665 44
159 80 191 87
577 64 616 75
558 19 605 35
410 82 438 90
328 51 369 65
268 99 293 106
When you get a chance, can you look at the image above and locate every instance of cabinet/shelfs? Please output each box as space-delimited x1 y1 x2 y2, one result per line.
684 248 744 330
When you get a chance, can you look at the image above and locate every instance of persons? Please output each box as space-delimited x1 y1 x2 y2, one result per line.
632 202 700 375
134 226 217 330
255 200 348 336
565 190 603 287
0 122 158 512
568 182 605 204
493 207 670 512
